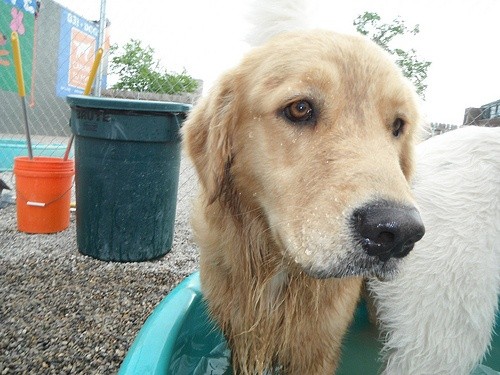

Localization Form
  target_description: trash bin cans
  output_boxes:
[63,95,192,264]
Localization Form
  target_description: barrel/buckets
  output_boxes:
[66,94,193,264]
[13,156,76,235]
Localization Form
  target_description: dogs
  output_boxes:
[180,28,427,375]
